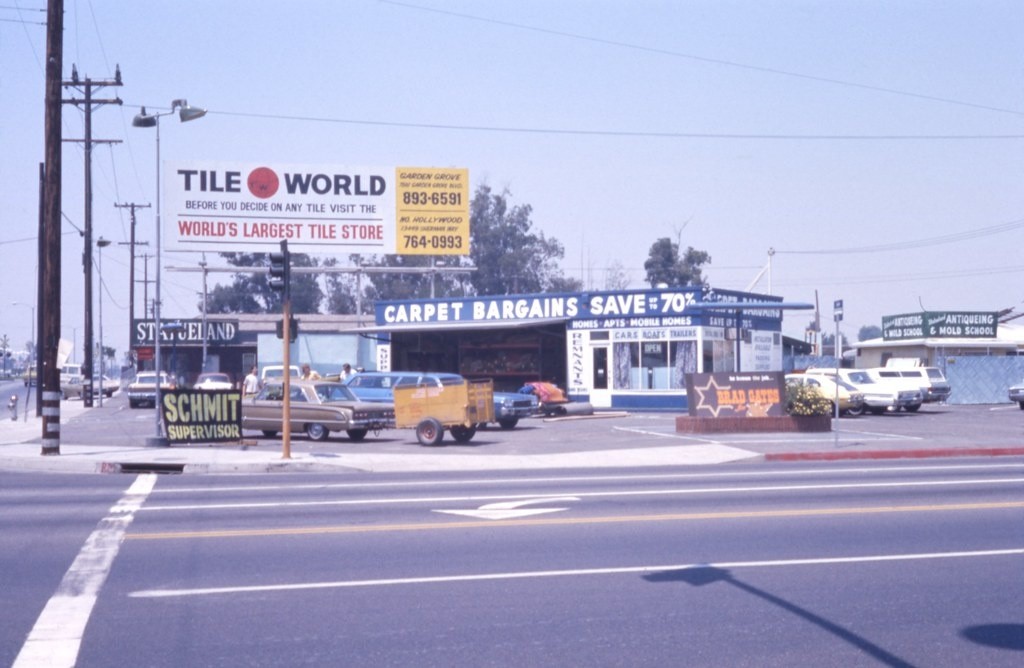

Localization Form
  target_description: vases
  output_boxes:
[787,413,836,432]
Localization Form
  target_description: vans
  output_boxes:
[60,363,89,384]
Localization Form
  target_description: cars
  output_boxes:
[59,375,118,400]
[191,371,235,395]
[126,370,174,407]
[22,364,37,388]
[1008,380,1024,411]
[241,380,393,440]
[784,373,864,416]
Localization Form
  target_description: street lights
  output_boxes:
[95,235,111,404]
[11,302,36,420]
[131,97,209,448]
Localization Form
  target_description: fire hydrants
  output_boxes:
[6,394,19,421]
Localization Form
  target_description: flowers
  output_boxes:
[784,376,834,417]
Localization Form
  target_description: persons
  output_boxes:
[300,363,321,382]
[339,363,361,387]
[519,384,536,394]
[357,368,368,385]
[242,365,260,396]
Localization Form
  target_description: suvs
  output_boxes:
[327,370,544,432]
[870,367,953,404]
[806,367,923,414]
